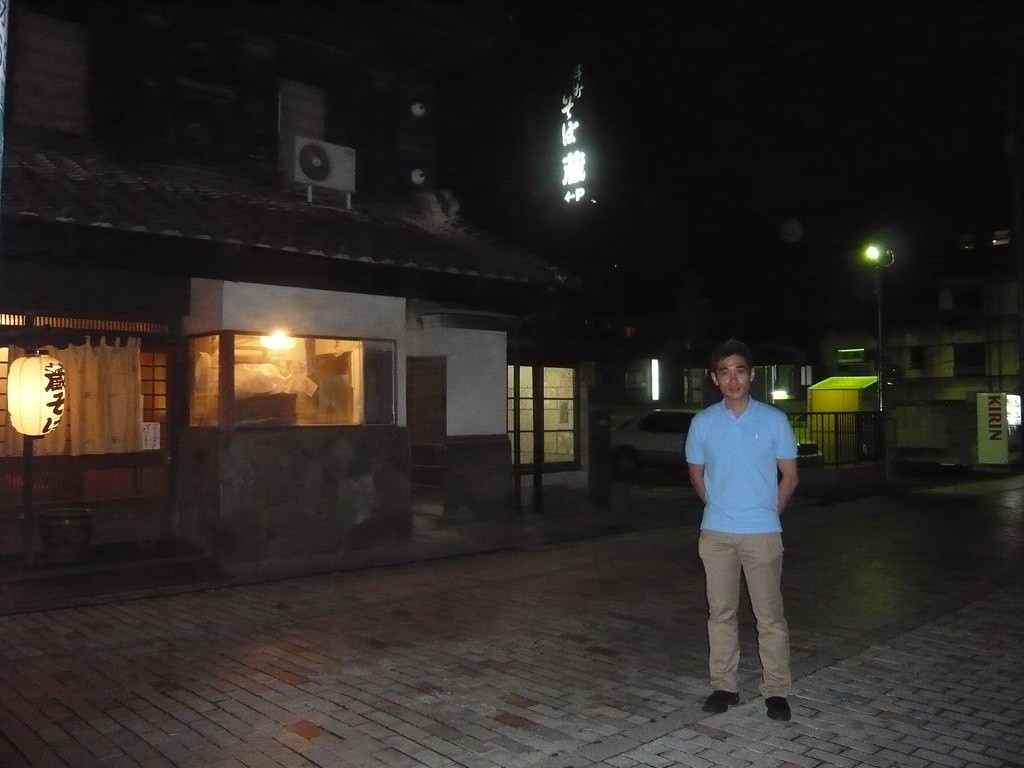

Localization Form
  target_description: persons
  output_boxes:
[685,339,799,720]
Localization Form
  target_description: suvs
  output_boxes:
[607,409,825,487]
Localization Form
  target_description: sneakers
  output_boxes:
[702,690,739,713]
[765,696,791,721]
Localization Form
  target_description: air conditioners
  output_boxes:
[289,134,355,195]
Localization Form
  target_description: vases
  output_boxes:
[39,505,93,561]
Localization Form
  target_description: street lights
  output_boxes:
[861,241,886,412]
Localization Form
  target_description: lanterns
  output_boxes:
[7,349,66,439]
